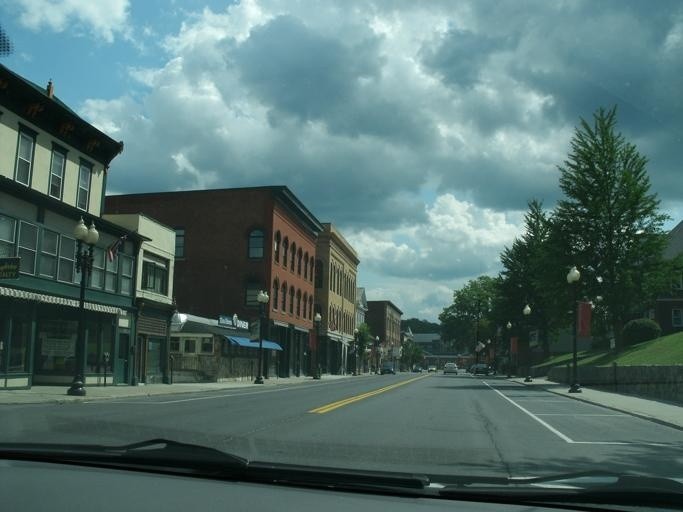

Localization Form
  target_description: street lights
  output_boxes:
[506,320,512,378]
[566,266,581,393]
[313,312,321,379]
[66,216,98,397]
[352,328,358,376]
[254,289,269,385]
[522,303,532,382]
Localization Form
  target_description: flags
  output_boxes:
[106,234,129,261]
[576,301,593,338]
[510,337,517,353]
[328,319,337,331]
[308,329,317,352]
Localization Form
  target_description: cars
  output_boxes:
[379,361,395,374]
[443,363,457,374]
[472,363,489,375]
[428,364,437,371]
[413,365,422,372]
